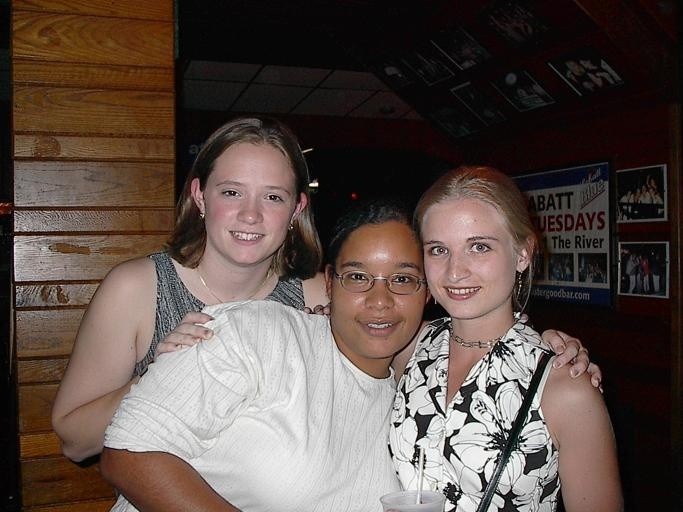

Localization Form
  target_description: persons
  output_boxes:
[104,200,609,512]
[49,115,340,502]
[299,164,630,512]
[617,174,666,297]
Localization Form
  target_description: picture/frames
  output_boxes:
[612,163,669,302]
[365,1,626,144]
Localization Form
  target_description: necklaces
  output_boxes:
[446,317,504,352]
[196,261,276,308]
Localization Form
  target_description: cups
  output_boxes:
[378,489,446,512]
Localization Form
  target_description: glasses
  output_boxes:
[329,266,427,295]
